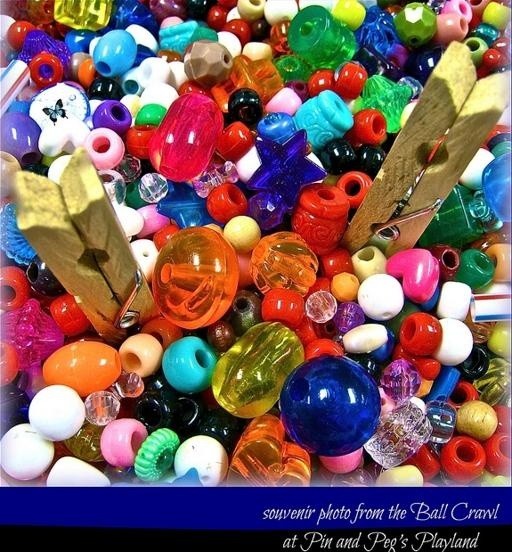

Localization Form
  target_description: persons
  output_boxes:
[0,1,512,485]
